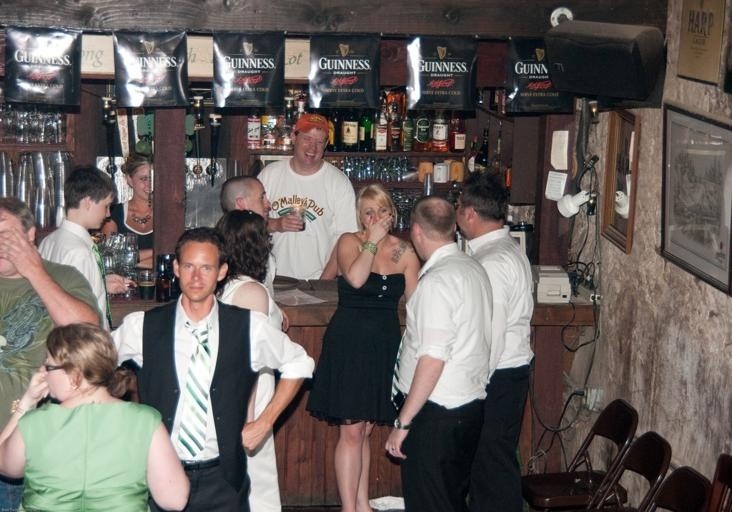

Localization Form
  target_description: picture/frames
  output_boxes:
[660,102,732,297]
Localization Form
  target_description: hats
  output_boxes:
[294,113,329,137]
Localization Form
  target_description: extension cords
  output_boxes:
[576,279,601,302]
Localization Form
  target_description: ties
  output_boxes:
[176,322,212,458]
[390,328,407,410]
[91,244,114,330]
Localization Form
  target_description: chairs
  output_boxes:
[519,397,638,512]
[647,466,711,512]
[590,431,672,512]
[703,455,732,512]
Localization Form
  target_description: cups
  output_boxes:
[98,233,140,299]
[138,270,157,300]
[339,156,462,230]
[2,107,67,231]
[157,252,177,301]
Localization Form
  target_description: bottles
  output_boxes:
[248,95,465,156]
[467,125,512,186]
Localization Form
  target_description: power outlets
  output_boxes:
[580,387,603,412]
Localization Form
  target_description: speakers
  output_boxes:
[545,20,664,101]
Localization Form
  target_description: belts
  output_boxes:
[496,365,530,374]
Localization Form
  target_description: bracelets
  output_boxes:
[364,241,378,254]
[13,407,28,416]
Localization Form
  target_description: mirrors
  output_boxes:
[600,109,642,257]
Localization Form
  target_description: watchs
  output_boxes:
[393,417,413,432]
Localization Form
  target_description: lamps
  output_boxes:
[556,154,598,218]
[614,190,631,220]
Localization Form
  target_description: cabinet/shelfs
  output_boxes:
[0,106,583,269]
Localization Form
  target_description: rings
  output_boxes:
[383,221,387,225]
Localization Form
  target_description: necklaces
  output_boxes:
[128,211,152,225]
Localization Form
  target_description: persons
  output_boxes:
[213,209,289,512]
[255,112,363,280]
[37,165,118,332]
[456,166,535,512]
[100,152,154,270]
[0,197,104,512]
[109,226,316,512]
[385,195,495,511]
[220,176,277,301]
[1,321,191,512]
[306,183,421,512]
